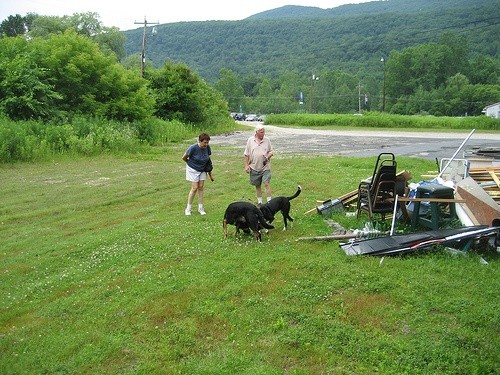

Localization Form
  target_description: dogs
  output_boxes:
[223,201,275,242]
[258,184,302,234]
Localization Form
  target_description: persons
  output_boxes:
[244,125,273,204]
[182,133,214,216]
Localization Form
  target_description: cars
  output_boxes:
[231,112,266,121]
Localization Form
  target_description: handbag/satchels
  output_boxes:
[198,159,213,172]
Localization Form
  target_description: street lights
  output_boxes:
[381,56,386,111]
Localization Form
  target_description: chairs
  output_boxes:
[357,153,397,223]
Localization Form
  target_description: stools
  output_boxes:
[411,183,456,232]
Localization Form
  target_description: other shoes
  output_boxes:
[198,208,206,215]
[257,203,263,208]
[185,209,191,215]
